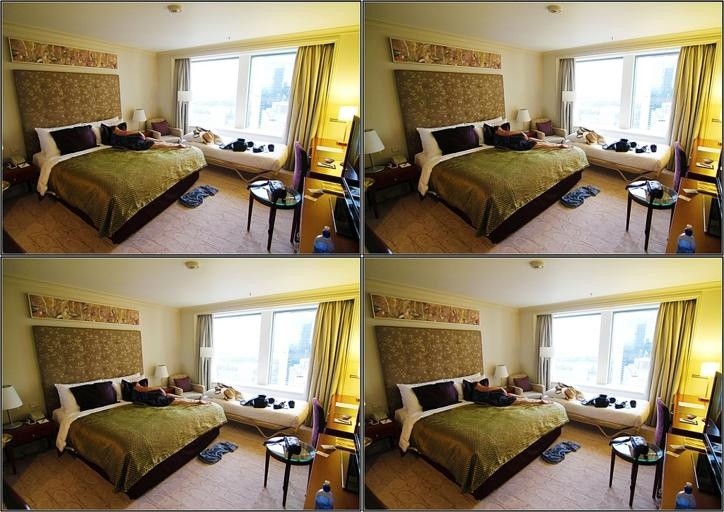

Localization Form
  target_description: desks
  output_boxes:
[665,138,721,254]
[661,394,721,510]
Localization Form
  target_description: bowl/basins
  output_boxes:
[323,158,334,164]
[340,414,351,421]
[703,158,713,164]
[685,414,697,420]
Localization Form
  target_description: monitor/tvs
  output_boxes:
[702,148,723,238]
[691,371,722,494]
[329,115,360,241]
[346,404,361,494]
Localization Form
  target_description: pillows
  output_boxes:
[183,130,206,143]
[152,120,171,136]
[37,114,128,161]
[415,116,511,161]
[568,131,589,144]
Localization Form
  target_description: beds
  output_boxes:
[626,180,678,251]
[32,141,590,511]
[562,129,672,183]
[543,389,651,440]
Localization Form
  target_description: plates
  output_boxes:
[334,417,353,426]
[317,161,336,170]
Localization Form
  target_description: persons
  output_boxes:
[108,124,188,152]
[491,123,569,152]
[469,380,552,407]
[128,380,209,407]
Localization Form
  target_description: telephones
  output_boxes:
[369,410,388,421]
[26,410,46,421]
[7,154,26,165]
[391,155,407,165]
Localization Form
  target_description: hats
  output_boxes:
[221,388,236,401]
[200,131,215,144]
[583,131,598,144]
[561,387,575,399]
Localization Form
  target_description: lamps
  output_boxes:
[698,361,720,402]
[516,109,531,132]
[364,129,386,175]
[131,109,147,133]
[336,104,356,146]
[3,384,24,430]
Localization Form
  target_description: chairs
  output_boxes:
[673,142,687,192]
[531,117,567,144]
[147,118,184,144]
[655,396,670,448]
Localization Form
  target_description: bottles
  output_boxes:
[314,480,334,509]
[675,481,698,509]
[313,226,335,253]
[676,225,697,254]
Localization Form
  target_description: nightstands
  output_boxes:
[3,157,36,201]
[3,415,55,474]
[524,129,537,138]
[143,131,153,137]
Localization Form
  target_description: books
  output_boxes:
[712,441,722,453]
[317,161,336,169]
[697,180,717,195]
[334,436,355,451]
[348,185,360,197]
[333,413,351,425]
[322,181,344,194]
[683,435,706,451]
